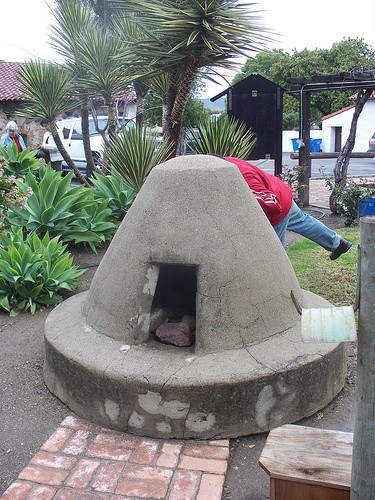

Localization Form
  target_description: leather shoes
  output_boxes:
[330,238,352,260]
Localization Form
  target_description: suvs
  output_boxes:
[43,116,141,180]
[182,127,207,155]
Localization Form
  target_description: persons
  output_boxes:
[0,121,27,153]
[209,154,352,260]
[38,118,65,172]
